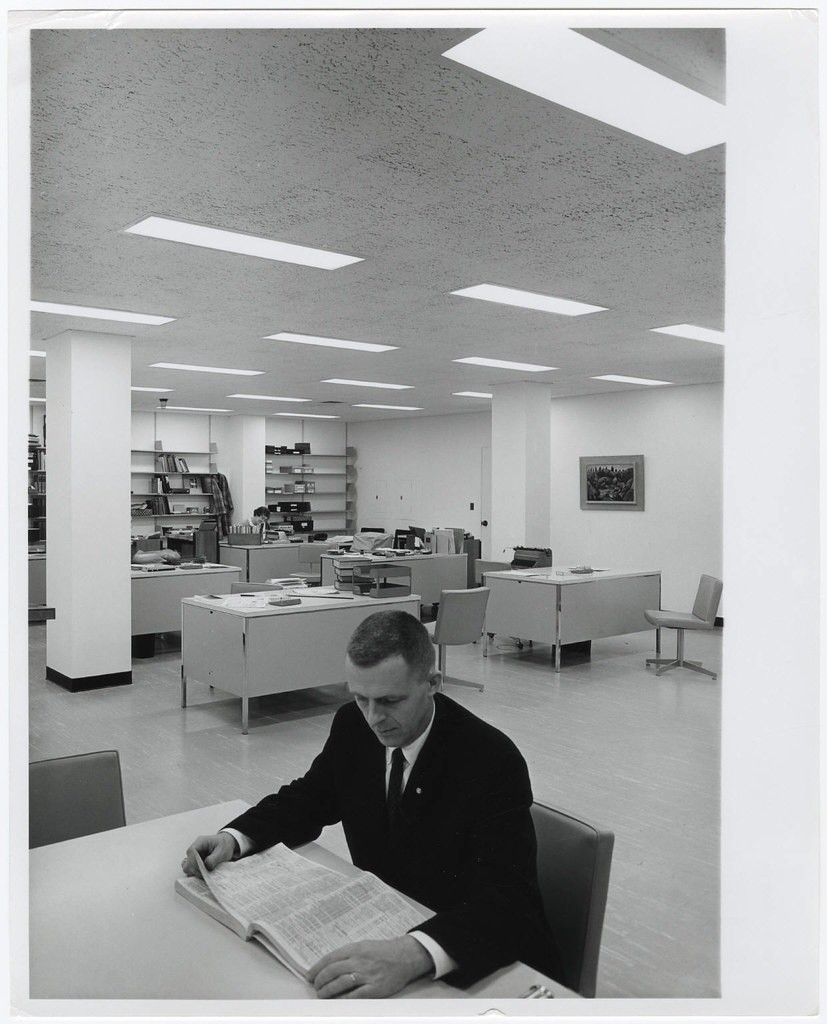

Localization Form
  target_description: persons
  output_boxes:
[180,610,552,999]
[131,516,180,563]
[240,506,271,540]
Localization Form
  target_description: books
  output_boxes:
[158,454,189,472]
[433,527,464,555]
[175,843,436,985]
[229,523,262,534]
[332,557,375,591]
[132,496,189,516]
[152,475,190,494]
[28,434,47,560]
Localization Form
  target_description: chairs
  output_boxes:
[424,587,490,692]
[30,750,125,848]
[474,558,524,649]
[291,543,332,587]
[643,574,723,680]
[230,583,283,596]
[349,532,393,551]
[531,799,615,999]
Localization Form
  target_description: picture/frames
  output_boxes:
[579,454,645,512]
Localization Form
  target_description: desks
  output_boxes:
[28,797,584,999]
[219,541,352,584]
[481,565,661,673]
[131,562,242,636]
[29,602,56,621]
[321,552,469,620]
[183,584,422,733]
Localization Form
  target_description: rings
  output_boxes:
[351,974,359,985]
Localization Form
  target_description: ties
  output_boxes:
[387,747,406,813]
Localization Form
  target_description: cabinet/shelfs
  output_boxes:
[129,440,218,519]
[28,447,46,606]
[268,452,356,533]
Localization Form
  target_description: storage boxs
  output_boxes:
[265,443,315,532]
[353,563,412,598]
